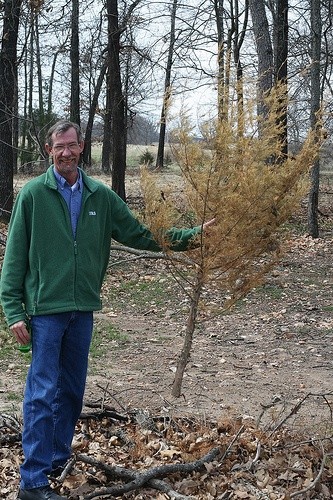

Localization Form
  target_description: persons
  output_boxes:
[0,119,216,500]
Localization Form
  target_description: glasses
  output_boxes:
[50,142,82,154]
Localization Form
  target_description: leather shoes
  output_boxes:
[19,485,66,500]
